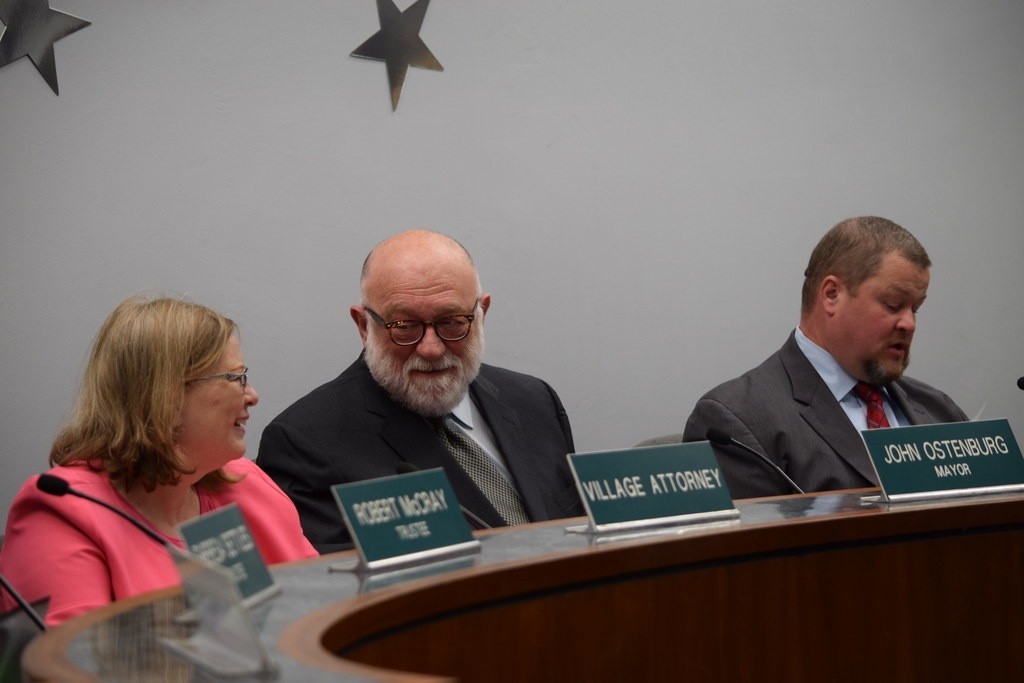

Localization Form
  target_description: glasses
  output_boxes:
[184,368,248,388]
[362,300,479,346]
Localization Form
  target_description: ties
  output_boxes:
[429,414,531,526]
[848,379,891,430]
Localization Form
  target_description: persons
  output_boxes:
[682,217,971,499]
[256,230,587,555]
[0,296,321,628]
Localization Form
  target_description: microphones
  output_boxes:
[397,463,493,531]
[1016,377,1024,391]
[706,427,805,496]
[36,474,169,547]
